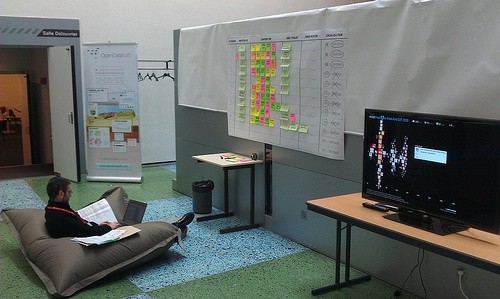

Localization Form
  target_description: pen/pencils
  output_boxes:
[220,153,251,164]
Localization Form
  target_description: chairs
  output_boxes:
[0,106,16,134]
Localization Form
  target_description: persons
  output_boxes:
[45,177,195,239]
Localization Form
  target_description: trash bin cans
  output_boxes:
[191,179,215,214]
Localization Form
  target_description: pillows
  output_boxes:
[0,185,185,299]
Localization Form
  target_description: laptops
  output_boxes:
[118,199,147,227]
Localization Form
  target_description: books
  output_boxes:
[76,198,117,225]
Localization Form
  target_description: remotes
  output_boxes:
[362,202,389,212]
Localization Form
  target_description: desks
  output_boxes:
[6,116,22,121]
[191,151,264,234]
[306,192,500,296]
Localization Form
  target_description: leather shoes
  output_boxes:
[172,212,195,229]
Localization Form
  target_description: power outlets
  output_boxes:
[454,260,467,281]
[300,208,308,223]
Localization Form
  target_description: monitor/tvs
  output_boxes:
[362,109,500,236]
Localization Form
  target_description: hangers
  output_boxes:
[138,70,174,82]
